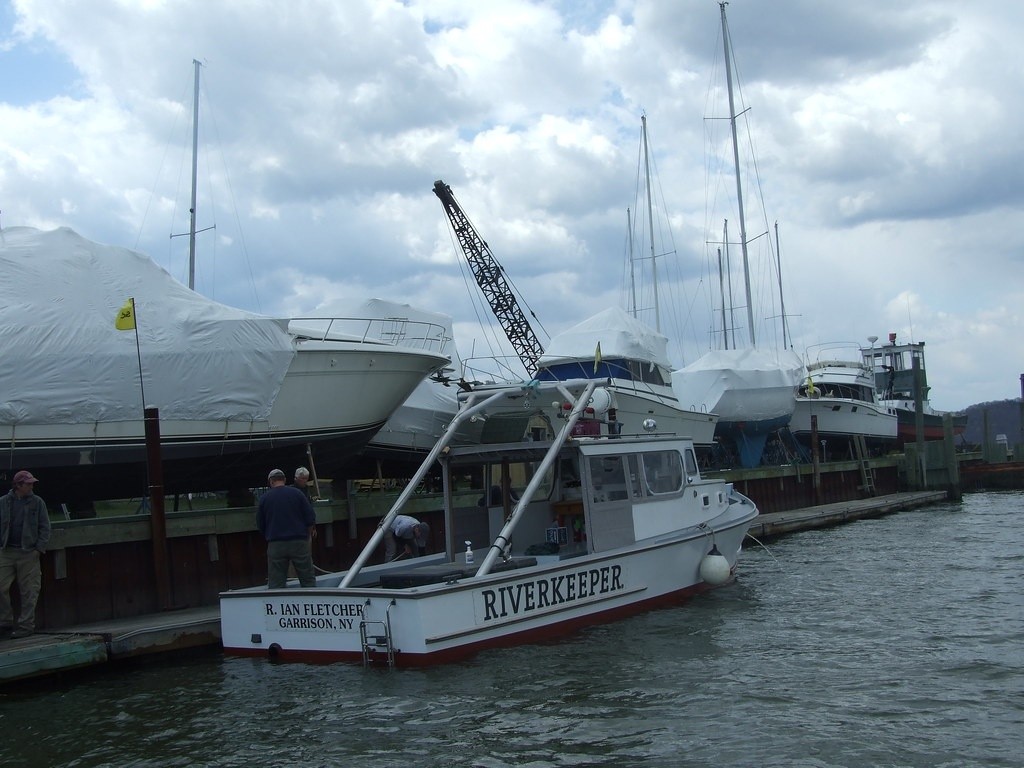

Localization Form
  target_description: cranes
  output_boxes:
[432,178,555,382]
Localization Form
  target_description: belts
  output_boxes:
[7,544,22,548]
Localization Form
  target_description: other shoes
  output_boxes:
[10,628,33,637]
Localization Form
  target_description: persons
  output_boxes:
[287,465,312,579]
[383,516,430,563]
[256,469,317,590]
[476,476,520,506]
[0,471,50,638]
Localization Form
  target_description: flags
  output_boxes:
[594,343,602,374]
[808,377,815,394]
[116,299,136,332]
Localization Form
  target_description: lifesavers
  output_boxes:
[807,386,821,399]
[556,514,586,541]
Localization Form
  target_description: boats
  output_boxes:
[0,292,971,522]
[217,351,761,675]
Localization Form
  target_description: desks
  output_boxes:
[554,501,584,527]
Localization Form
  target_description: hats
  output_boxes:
[419,522,430,541]
[14,471,40,483]
[268,469,284,480]
